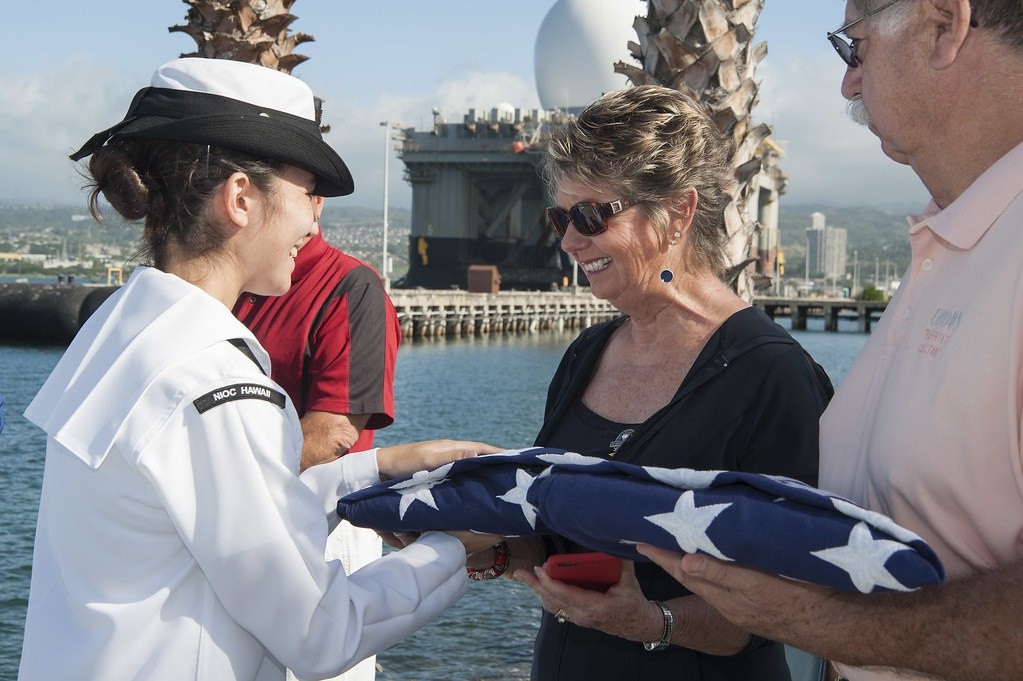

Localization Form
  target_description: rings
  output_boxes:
[554,608,568,624]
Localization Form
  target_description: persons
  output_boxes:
[377,85,834,681]
[637,1,1023,681]
[15,56,506,681]
[232,194,402,681]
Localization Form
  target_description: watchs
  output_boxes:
[640,600,674,652]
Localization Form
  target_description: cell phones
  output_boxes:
[545,552,623,594]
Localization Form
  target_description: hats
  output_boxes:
[68,57,355,197]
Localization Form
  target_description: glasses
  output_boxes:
[828,0,979,68]
[545,195,643,238]
